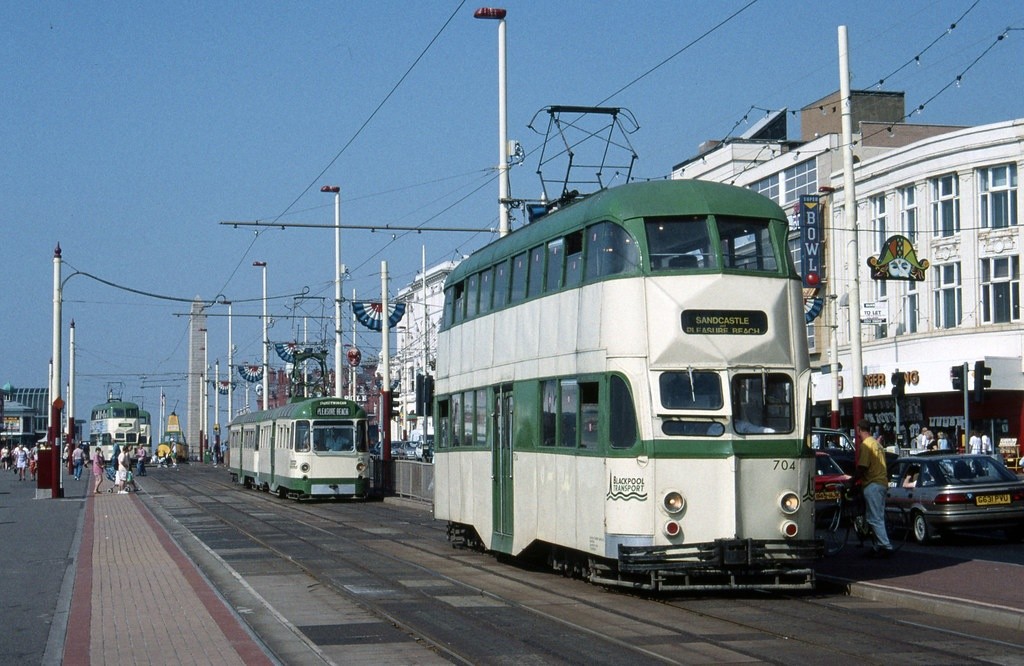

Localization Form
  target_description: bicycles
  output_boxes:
[816,483,916,559]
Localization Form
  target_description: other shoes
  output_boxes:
[75,476,77,480]
[874,549,894,560]
[94,491,100,494]
[134,488,141,491]
[19,479,22,481]
[863,551,876,559]
[117,489,129,494]
[77,478,79,480]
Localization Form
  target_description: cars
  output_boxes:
[882,453,1024,545]
[366,426,434,462]
[812,446,852,517]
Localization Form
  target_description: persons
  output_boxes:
[325,430,352,450]
[134,444,147,476]
[213,441,220,465]
[0,443,51,480]
[92,439,142,494]
[968,430,991,454]
[154,438,177,467]
[849,421,893,550]
[706,419,775,435]
[903,464,937,488]
[911,428,954,450]
[64,441,89,480]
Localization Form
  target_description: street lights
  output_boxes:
[218,300,234,423]
[320,184,343,398]
[198,328,209,452]
[252,261,269,411]
[472,7,512,238]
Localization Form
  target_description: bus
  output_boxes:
[434,103,826,597]
[131,395,153,464]
[226,347,373,500]
[87,380,141,466]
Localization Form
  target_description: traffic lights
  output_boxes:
[891,371,907,397]
[950,363,965,390]
[390,389,402,417]
[974,360,992,403]
